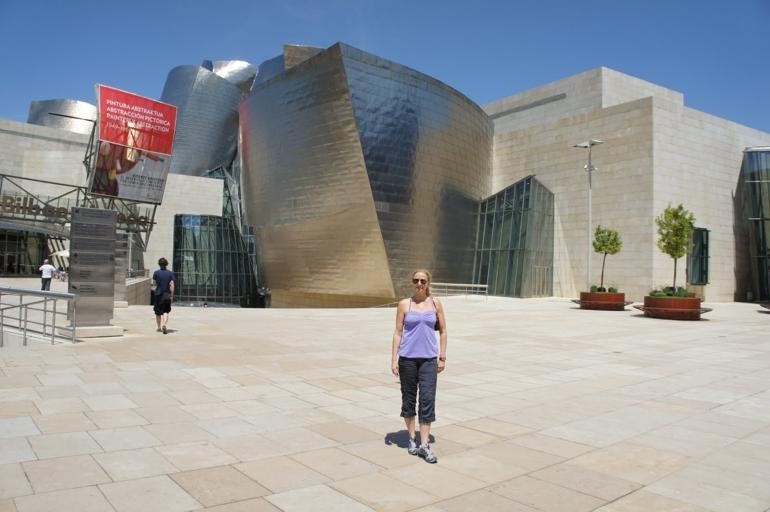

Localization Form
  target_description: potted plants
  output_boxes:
[643,201,701,320]
[581,223,624,311]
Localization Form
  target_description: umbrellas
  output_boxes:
[48,249,70,257]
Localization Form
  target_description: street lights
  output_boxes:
[572,138,606,293]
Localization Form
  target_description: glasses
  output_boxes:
[413,279,426,284]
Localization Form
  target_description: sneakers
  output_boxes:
[157,326,167,334]
[408,438,437,463]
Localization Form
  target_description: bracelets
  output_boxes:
[170,296,174,297]
[439,357,446,361]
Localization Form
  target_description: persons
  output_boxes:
[151,258,175,334]
[391,269,447,463]
[56,267,69,281]
[39,259,56,291]
